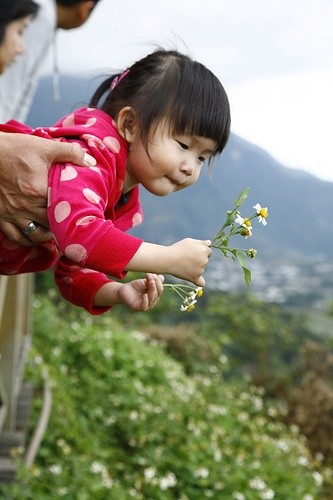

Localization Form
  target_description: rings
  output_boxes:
[22,221,40,237]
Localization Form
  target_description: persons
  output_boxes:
[0,51,232,316]
[0,0,102,247]
